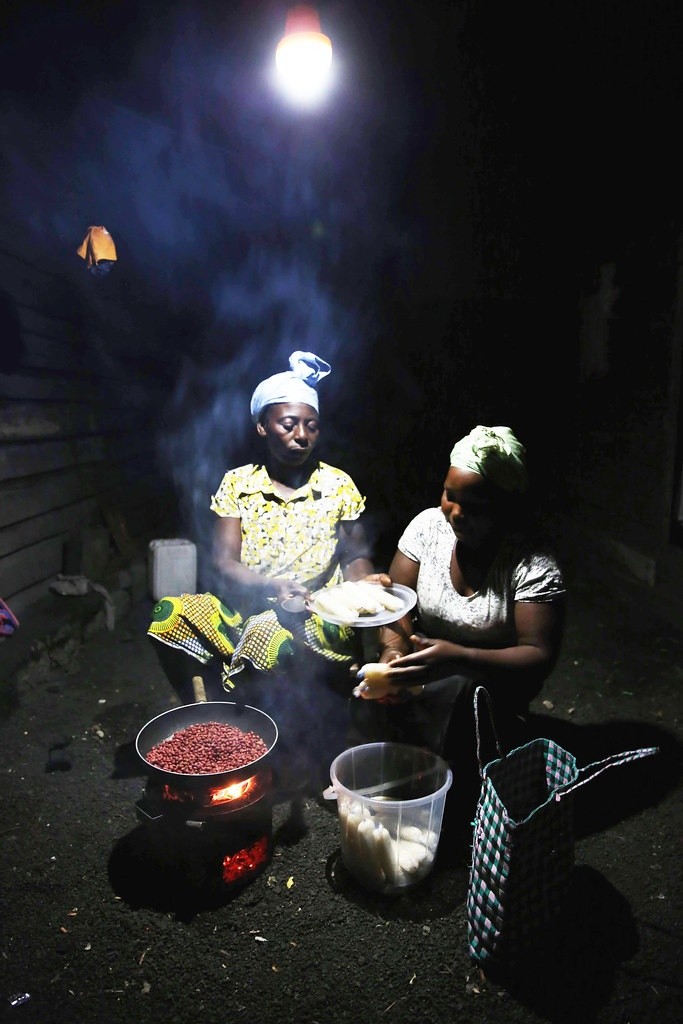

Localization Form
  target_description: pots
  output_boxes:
[133,674,279,800]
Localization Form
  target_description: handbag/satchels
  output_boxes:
[467,684,662,966]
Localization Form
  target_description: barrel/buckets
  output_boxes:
[322,740,454,893]
[147,536,198,602]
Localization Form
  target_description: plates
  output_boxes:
[305,579,418,629]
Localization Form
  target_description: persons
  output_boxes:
[323,424,567,801]
[147,350,392,794]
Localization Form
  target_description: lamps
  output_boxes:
[273,0,335,107]
